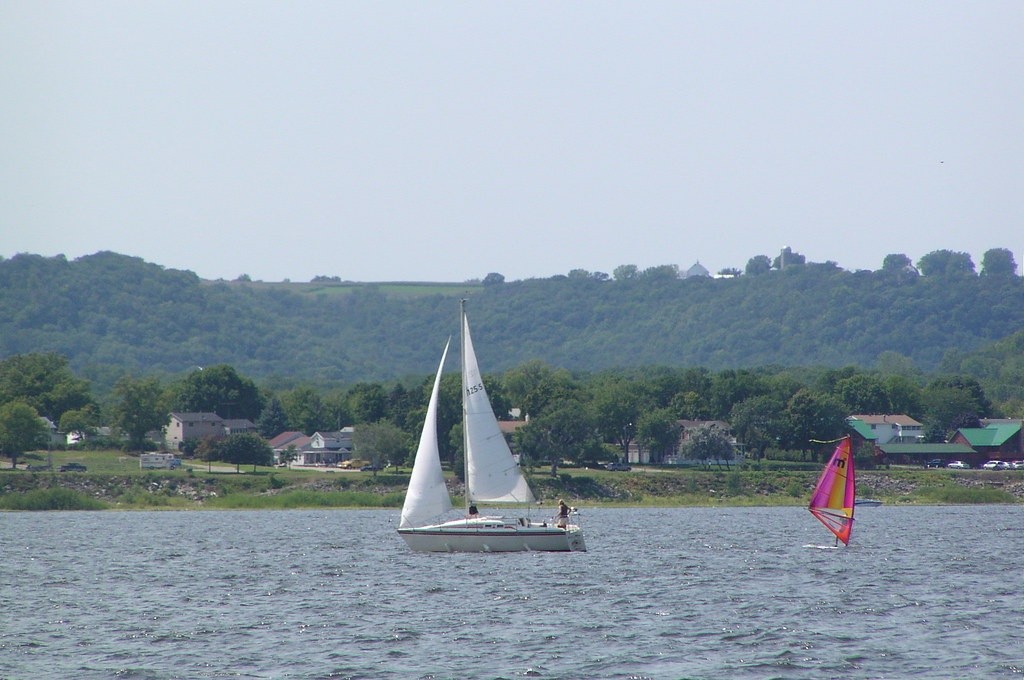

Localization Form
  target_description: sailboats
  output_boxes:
[801,434,856,549]
[396,295,589,553]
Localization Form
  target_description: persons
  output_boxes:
[469,501,478,519]
[552,500,571,530]
[835,513,847,547]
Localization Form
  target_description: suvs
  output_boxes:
[607,462,632,472]
[927,458,946,468]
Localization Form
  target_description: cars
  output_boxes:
[947,461,970,469]
[983,460,1024,471]
[360,462,383,471]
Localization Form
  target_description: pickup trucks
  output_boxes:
[341,457,369,470]
[57,462,87,472]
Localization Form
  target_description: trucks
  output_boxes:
[139,454,175,469]
